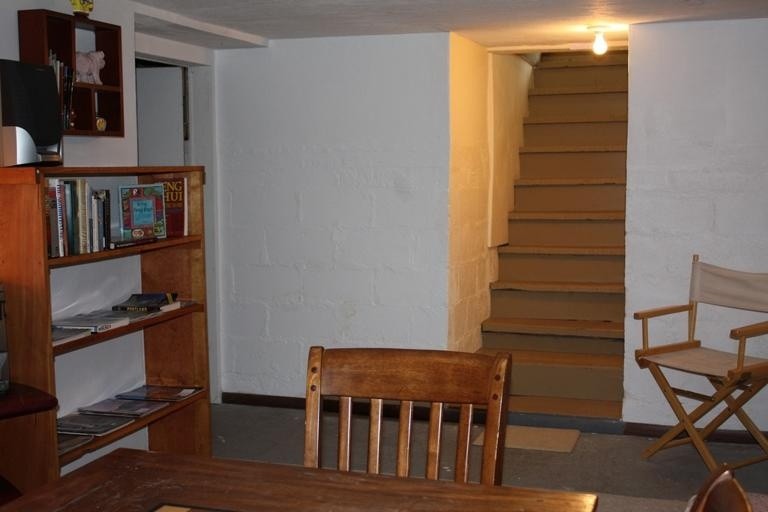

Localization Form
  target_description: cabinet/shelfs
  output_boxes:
[15,10,126,139]
[1,163,212,500]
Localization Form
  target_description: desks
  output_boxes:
[0,446,599,512]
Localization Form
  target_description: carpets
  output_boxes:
[472,417,581,455]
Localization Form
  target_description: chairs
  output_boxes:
[632,253,768,477]
[298,342,510,489]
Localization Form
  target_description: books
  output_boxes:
[57,385,204,457]
[52,293,180,347]
[48,49,76,129]
[44,177,190,258]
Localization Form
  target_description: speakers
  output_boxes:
[0,60,63,168]
